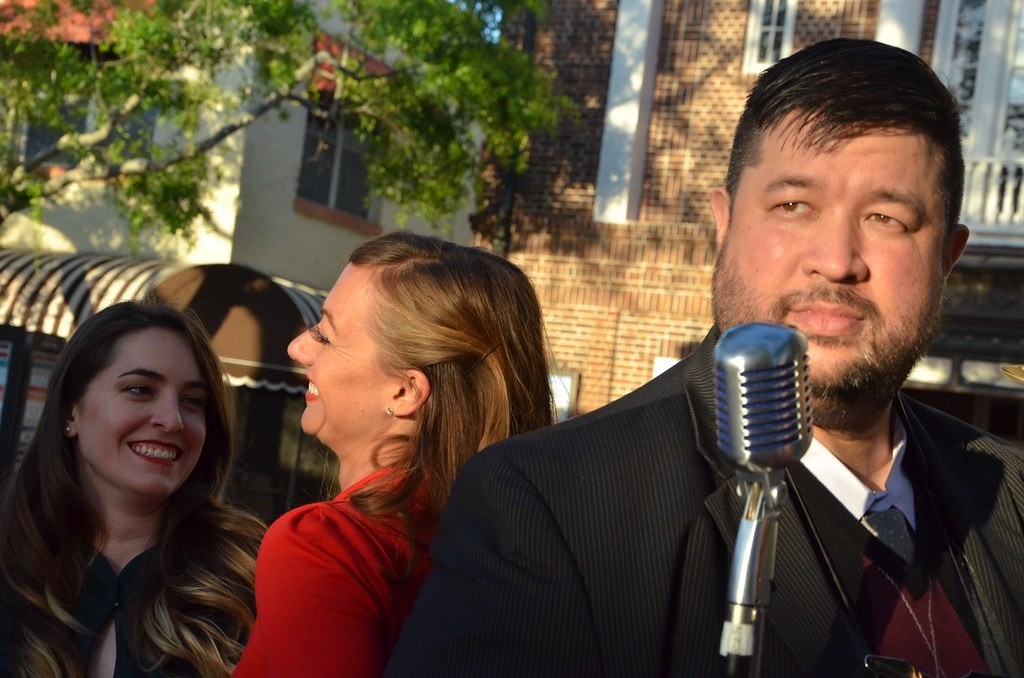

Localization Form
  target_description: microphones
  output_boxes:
[711,320,813,678]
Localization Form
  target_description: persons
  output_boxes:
[391,36,1024,678]
[0,298,267,678]
[231,228,558,678]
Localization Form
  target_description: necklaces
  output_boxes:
[876,566,949,678]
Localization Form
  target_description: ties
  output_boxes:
[860,503,912,566]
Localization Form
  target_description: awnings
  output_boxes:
[0,245,326,395]
